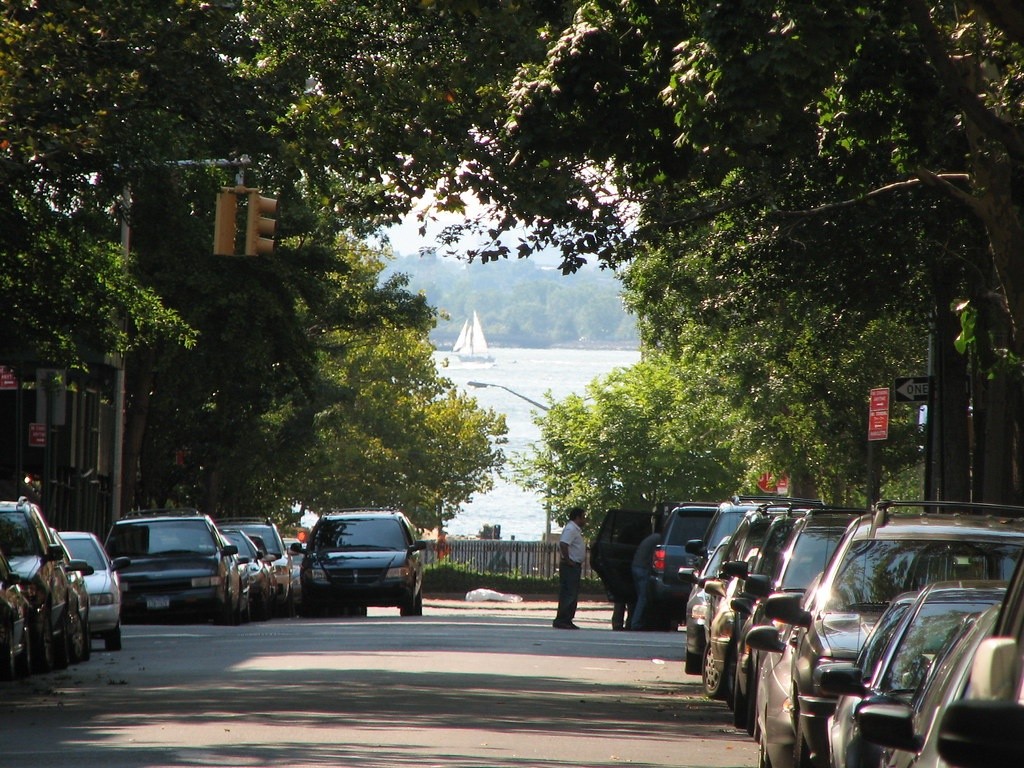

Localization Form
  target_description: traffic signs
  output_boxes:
[893,375,927,402]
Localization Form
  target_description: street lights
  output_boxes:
[468,380,554,543]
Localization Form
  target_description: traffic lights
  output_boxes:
[244,192,278,257]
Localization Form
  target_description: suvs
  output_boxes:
[0,497,92,679]
[290,506,429,617]
[587,498,720,621]
[679,495,1023,768]
[101,508,245,627]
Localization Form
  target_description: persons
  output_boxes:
[553,507,586,629]
[612,532,662,631]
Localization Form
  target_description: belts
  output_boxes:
[561,558,581,564]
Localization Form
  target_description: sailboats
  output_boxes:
[451,312,496,365]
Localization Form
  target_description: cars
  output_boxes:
[209,515,310,619]
[57,529,131,652]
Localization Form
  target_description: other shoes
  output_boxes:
[626,625,631,630]
[569,621,580,629]
[553,619,573,629]
[613,626,625,630]
